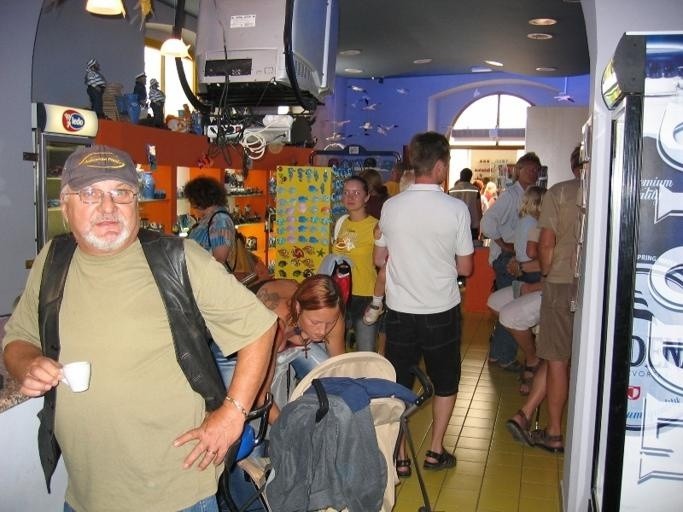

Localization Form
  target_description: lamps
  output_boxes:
[86,0,125,17]
[159,0,192,59]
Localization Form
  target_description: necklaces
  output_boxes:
[292,309,313,360]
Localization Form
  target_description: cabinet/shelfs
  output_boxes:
[221,168,268,267]
[175,163,224,234]
[136,164,174,231]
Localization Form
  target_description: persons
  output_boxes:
[0,143,282,512]
[84,57,105,118]
[332,132,584,478]
[148,79,166,128]
[179,180,245,275]
[210,278,347,439]
[132,73,148,122]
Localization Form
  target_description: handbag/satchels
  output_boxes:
[207,210,257,286]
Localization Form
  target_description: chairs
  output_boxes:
[220,392,276,511]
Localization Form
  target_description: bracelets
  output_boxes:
[224,395,249,425]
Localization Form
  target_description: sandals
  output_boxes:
[396,458,411,476]
[531,429,564,452]
[506,409,536,448]
[424,447,456,468]
[520,357,544,396]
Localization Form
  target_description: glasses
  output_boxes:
[341,190,360,198]
[64,189,140,204]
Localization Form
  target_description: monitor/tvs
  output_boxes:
[194,0,340,105]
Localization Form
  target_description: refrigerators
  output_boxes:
[27,99,99,256]
[590,29,683,511]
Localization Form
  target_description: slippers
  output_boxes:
[498,360,523,372]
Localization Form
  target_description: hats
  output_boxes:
[60,144,140,192]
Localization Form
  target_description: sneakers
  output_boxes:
[362,301,385,326]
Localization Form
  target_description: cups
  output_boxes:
[60,361,90,394]
